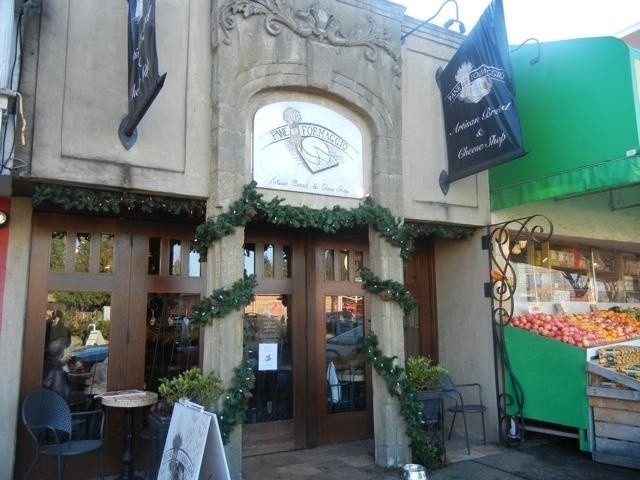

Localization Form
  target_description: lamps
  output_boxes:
[400,0,466,46]
[511,38,541,65]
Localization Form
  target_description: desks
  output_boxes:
[101,389,158,479]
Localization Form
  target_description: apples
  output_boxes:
[590,313,638,339]
[508,312,606,347]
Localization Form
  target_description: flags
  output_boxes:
[124,0,162,135]
[439,0,525,185]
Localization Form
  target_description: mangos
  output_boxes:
[605,324,625,342]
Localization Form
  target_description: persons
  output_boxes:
[45,310,72,372]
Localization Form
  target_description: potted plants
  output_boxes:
[150,365,225,480]
[405,354,448,425]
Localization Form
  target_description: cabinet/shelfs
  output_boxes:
[508,232,640,303]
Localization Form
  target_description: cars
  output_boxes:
[325,324,364,370]
[68,344,110,374]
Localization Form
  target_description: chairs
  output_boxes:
[21,387,107,480]
[429,371,486,455]
